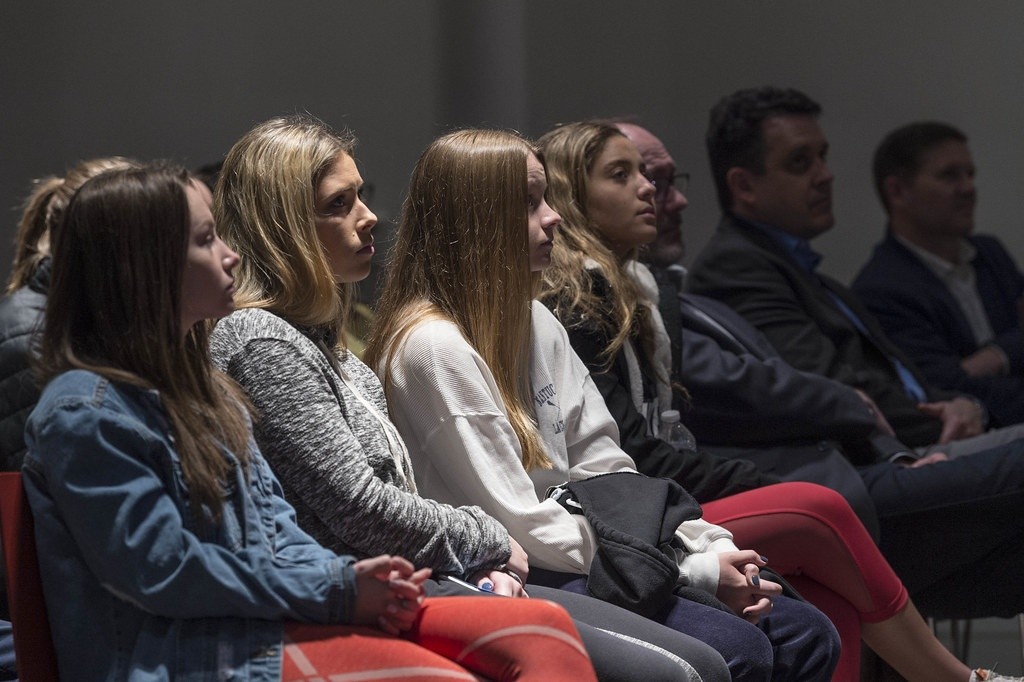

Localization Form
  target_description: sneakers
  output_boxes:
[968,665,1022,682]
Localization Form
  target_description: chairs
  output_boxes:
[0,472,58,682]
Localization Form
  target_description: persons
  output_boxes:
[358,125,843,682]
[200,114,735,682]
[20,161,602,681]
[528,120,1024,682]
[844,121,1024,431]
[1,157,234,482]
[605,124,1024,620]
[689,83,1024,449]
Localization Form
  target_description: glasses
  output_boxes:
[652,167,692,201]
